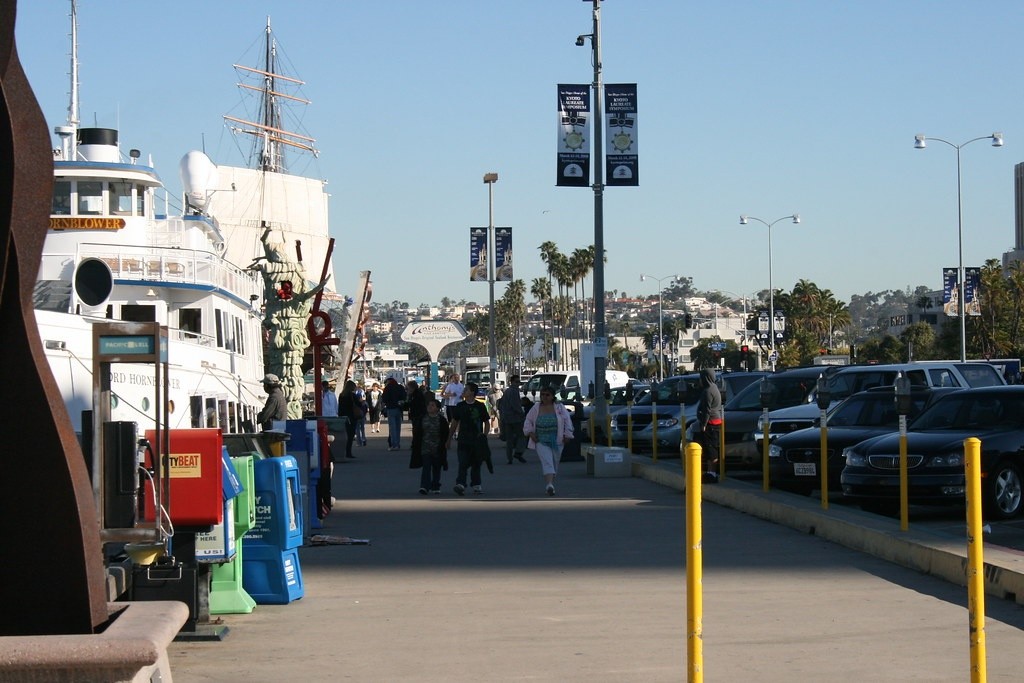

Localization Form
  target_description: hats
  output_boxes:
[259,373,281,385]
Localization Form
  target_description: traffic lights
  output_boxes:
[741,345,750,361]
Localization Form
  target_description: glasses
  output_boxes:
[463,387,471,392]
[540,392,552,397]
[514,380,520,384]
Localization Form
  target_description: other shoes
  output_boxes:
[544,483,557,497]
[418,487,429,496]
[377,428,381,433]
[355,443,361,447]
[346,453,355,457]
[362,441,367,446]
[507,459,513,464]
[513,452,527,463]
[370,428,376,434]
[702,472,719,484]
[431,490,441,495]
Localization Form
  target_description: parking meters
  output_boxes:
[893,369,912,531]
[815,370,831,510]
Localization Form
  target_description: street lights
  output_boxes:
[707,288,783,346]
[914,132,1004,363]
[738,213,801,372]
[640,273,681,382]
[480,172,502,391]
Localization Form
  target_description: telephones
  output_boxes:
[101,420,158,493]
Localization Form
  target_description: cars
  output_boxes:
[840,385,1024,521]
[601,357,1022,471]
[358,378,381,387]
[769,385,1003,496]
[438,369,534,397]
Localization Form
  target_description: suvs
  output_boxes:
[753,362,1008,461]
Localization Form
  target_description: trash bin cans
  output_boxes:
[142,427,220,622]
[194,444,244,622]
[243,453,305,604]
[209,455,257,614]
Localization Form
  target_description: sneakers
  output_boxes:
[387,446,401,452]
[472,484,483,492]
[453,484,466,496]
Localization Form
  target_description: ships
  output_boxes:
[24,0,347,488]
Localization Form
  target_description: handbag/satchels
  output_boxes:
[708,417,723,427]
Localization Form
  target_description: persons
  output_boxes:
[256,374,288,457]
[320,373,573,496]
[696,369,723,484]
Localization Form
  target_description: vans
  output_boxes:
[517,370,630,406]
